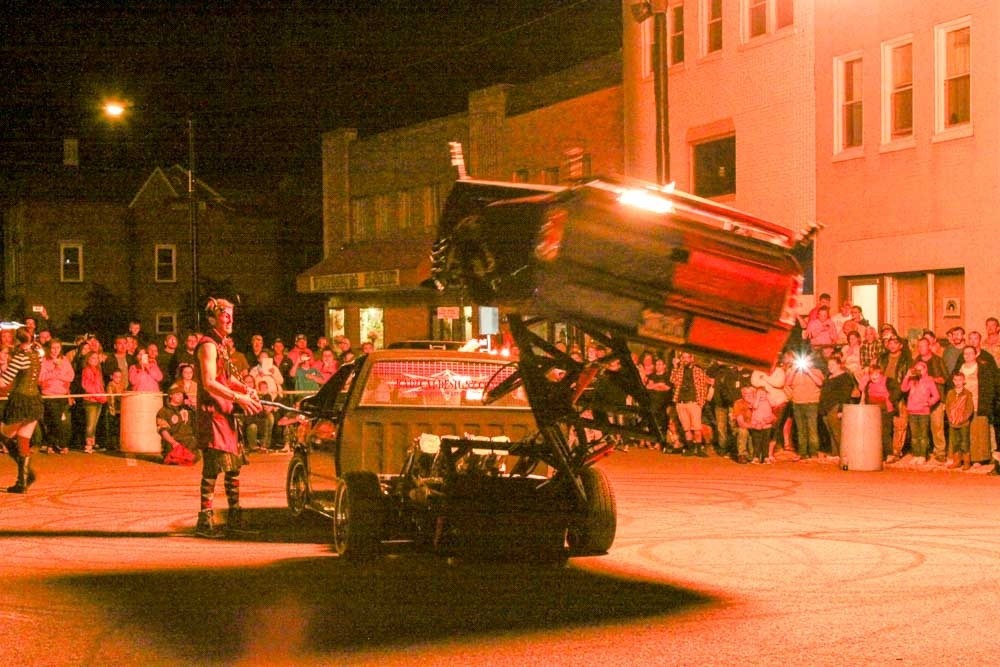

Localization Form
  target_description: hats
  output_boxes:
[169,388,189,400]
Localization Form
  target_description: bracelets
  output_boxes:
[232,393,239,402]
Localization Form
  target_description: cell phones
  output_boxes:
[32,306,43,312]
[911,370,921,374]
[141,354,148,364]
[301,356,309,360]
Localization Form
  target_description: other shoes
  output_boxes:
[84,444,92,454]
[917,456,927,465]
[60,447,69,455]
[886,455,896,463]
[718,447,728,456]
[935,455,946,464]
[973,461,982,468]
[750,457,760,464]
[909,455,919,464]
[47,447,55,454]
[763,457,771,465]
[738,455,747,464]
[988,462,1000,475]
[948,463,962,469]
[963,463,971,470]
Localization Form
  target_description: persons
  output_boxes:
[191,301,263,537]
[157,332,374,464]
[0,317,163,454]
[0,327,42,492]
[553,332,825,463]
[792,292,1000,475]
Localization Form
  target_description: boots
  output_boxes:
[196,510,225,537]
[8,440,35,486]
[223,508,263,534]
[7,456,30,493]
[684,440,695,456]
[696,443,709,457]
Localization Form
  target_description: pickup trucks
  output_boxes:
[279,140,830,557]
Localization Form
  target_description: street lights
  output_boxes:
[632,0,671,185]
[97,94,201,340]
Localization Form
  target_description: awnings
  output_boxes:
[295,240,436,292]
